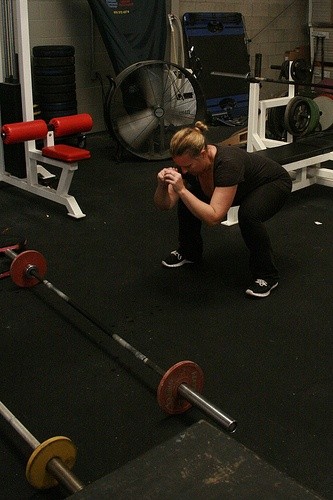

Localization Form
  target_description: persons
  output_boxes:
[151,120,293,298]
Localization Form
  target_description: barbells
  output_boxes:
[4,246,240,433]
[1,400,84,493]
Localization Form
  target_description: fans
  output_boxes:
[104,59,207,160]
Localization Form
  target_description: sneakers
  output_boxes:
[246,273,279,298]
[162,250,196,267]
